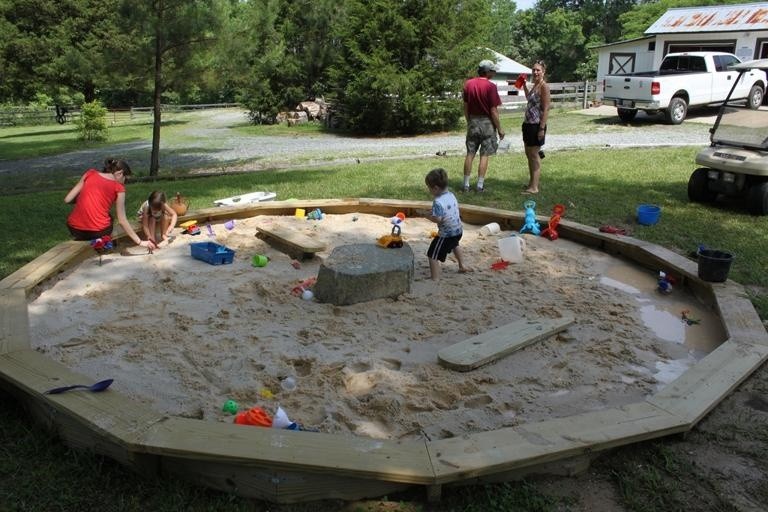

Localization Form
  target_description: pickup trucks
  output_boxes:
[600,50,767,125]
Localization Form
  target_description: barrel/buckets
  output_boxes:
[696,246,737,283]
[636,204,662,225]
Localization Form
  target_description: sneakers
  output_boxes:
[463,185,488,193]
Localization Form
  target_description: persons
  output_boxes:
[63,157,156,249]
[459,60,505,193]
[516,60,551,195]
[422,167,474,282]
[137,190,178,247]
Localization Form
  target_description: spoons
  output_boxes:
[43,378,114,399]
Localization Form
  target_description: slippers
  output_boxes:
[521,190,534,194]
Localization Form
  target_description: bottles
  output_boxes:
[697,239,705,255]
[392,217,403,225]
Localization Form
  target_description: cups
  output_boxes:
[515,71,525,90]
[252,254,268,267]
[295,208,306,218]
[225,220,235,230]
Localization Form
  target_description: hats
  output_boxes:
[478,60,500,70]
